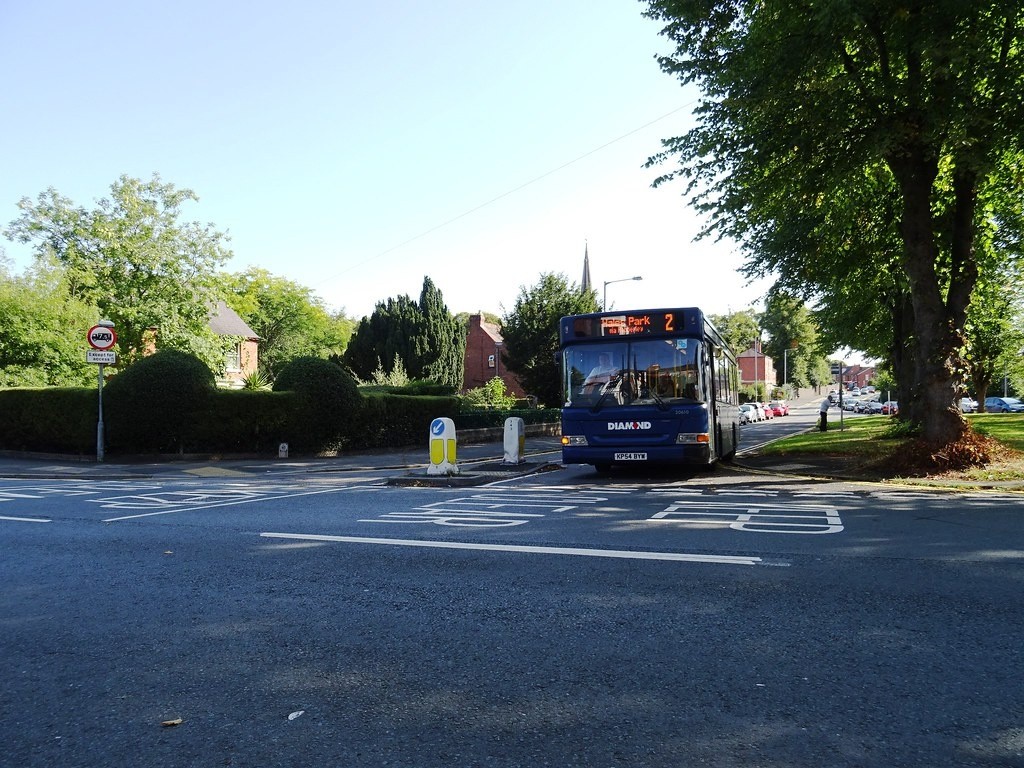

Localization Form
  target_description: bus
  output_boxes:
[555,306,740,477]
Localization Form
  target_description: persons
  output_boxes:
[615,371,641,398]
[653,375,675,397]
[820,396,839,432]
[585,354,618,383]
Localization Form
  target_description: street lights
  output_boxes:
[782,346,798,385]
[602,275,644,311]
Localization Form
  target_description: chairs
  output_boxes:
[652,379,676,398]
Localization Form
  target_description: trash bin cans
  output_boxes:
[499,417,527,464]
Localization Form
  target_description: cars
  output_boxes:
[961,398,980,413]
[829,384,898,415]
[738,406,748,426]
[740,405,758,423]
[779,402,789,416]
[770,403,784,417]
[984,396,1024,413]
[762,403,773,420]
[743,402,766,420]
